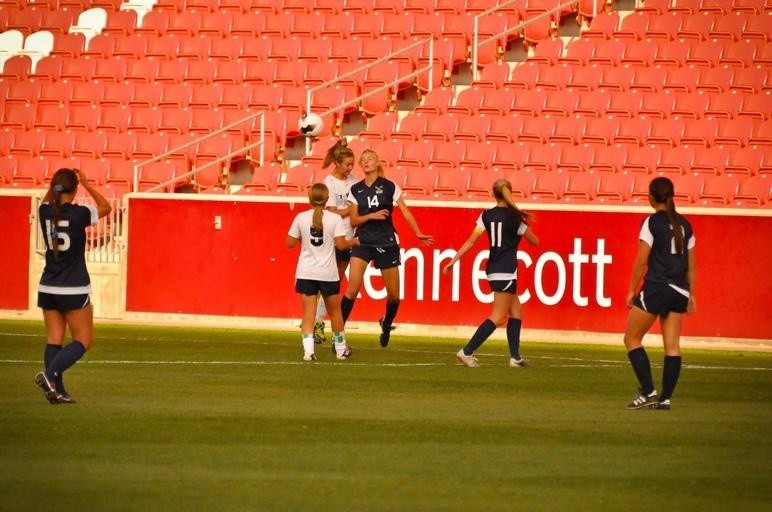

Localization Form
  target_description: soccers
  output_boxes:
[298,114,324,136]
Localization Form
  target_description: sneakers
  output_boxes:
[299,322,326,361]
[379,317,391,346]
[332,338,352,360]
[510,356,529,368]
[626,390,658,410]
[650,398,670,410]
[457,349,480,368]
[34,372,74,403]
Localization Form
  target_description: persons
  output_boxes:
[34,168,112,405]
[443,179,540,367]
[332,149,437,353]
[298,138,358,345]
[623,177,697,410]
[286,183,362,361]
